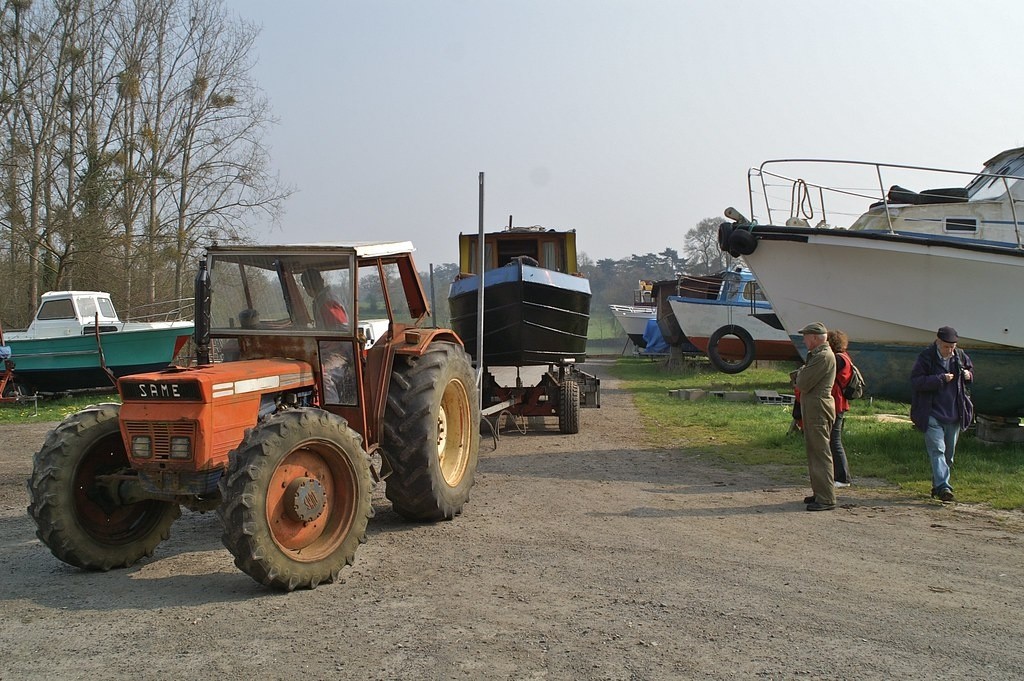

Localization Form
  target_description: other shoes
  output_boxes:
[804,496,835,511]
[931,488,953,500]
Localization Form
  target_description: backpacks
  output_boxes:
[834,352,866,400]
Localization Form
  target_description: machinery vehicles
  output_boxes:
[26,242,482,593]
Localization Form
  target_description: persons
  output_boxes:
[792,330,852,488]
[301,268,354,404]
[911,326,973,500]
[795,322,836,511]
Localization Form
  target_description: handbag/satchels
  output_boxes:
[957,347,976,426]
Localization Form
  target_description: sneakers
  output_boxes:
[834,482,850,487]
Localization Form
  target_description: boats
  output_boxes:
[0,290,195,399]
[446,215,591,367]
[666,264,803,362]
[717,147,1024,417]
[609,290,701,353]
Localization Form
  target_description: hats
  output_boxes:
[797,322,827,334]
[937,326,958,343]
